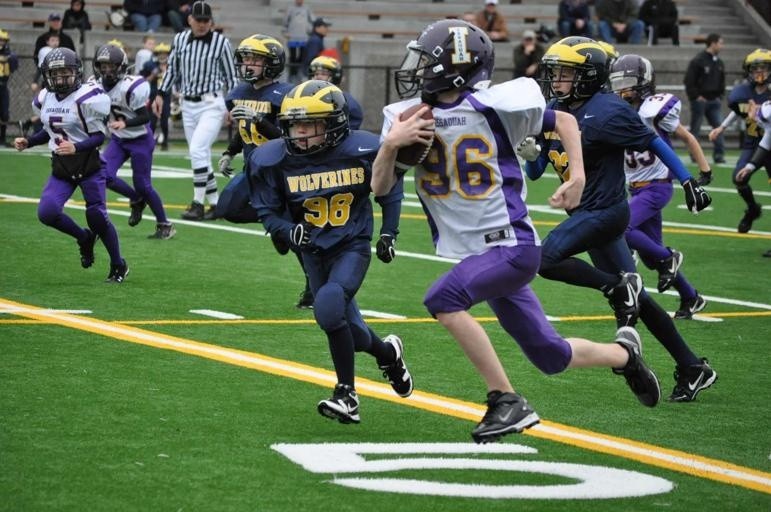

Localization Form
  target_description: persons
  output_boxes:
[282,1,332,82]
[218,18,718,441]
[473,0,680,46]
[684,34,771,257]
[1,0,240,282]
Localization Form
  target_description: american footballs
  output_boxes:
[394,104,433,177]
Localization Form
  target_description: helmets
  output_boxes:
[536,36,655,103]
[233,33,349,157]
[41,44,128,93]
[742,48,771,86]
[395,19,495,99]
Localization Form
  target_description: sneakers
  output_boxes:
[376,334,414,398]
[182,201,223,220]
[147,223,177,240]
[77,228,99,268]
[104,258,129,284]
[471,389,540,444]
[738,204,762,234]
[317,384,360,424]
[604,247,718,407]
[128,195,146,226]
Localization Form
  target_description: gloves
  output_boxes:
[288,222,320,254]
[683,170,714,216]
[376,233,396,263]
[229,105,262,124]
[218,154,235,178]
[516,137,541,162]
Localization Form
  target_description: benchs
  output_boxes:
[1,0,710,44]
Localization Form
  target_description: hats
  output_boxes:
[192,1,212,20]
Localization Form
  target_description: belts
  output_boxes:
[184,93,217,101]
